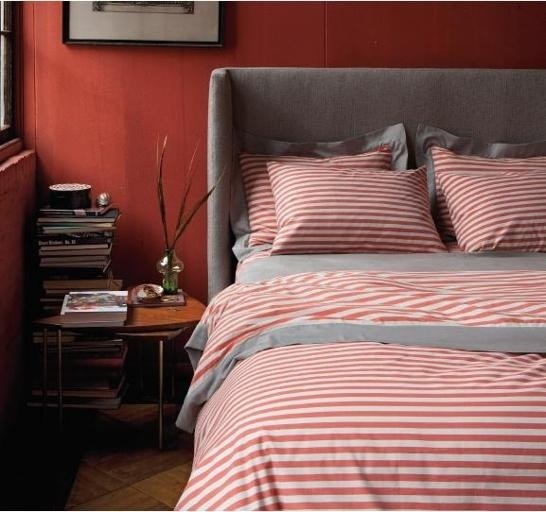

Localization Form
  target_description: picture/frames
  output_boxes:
[60,2,227,49]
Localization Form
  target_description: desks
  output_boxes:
[33,281,208,453]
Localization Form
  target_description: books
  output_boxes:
[26,204,128,409]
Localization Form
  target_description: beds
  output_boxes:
[199,66,546,510]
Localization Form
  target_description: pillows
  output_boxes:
[228,122,449,264]
[414,123,546,262]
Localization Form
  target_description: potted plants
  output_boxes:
[153,134,228,295]
